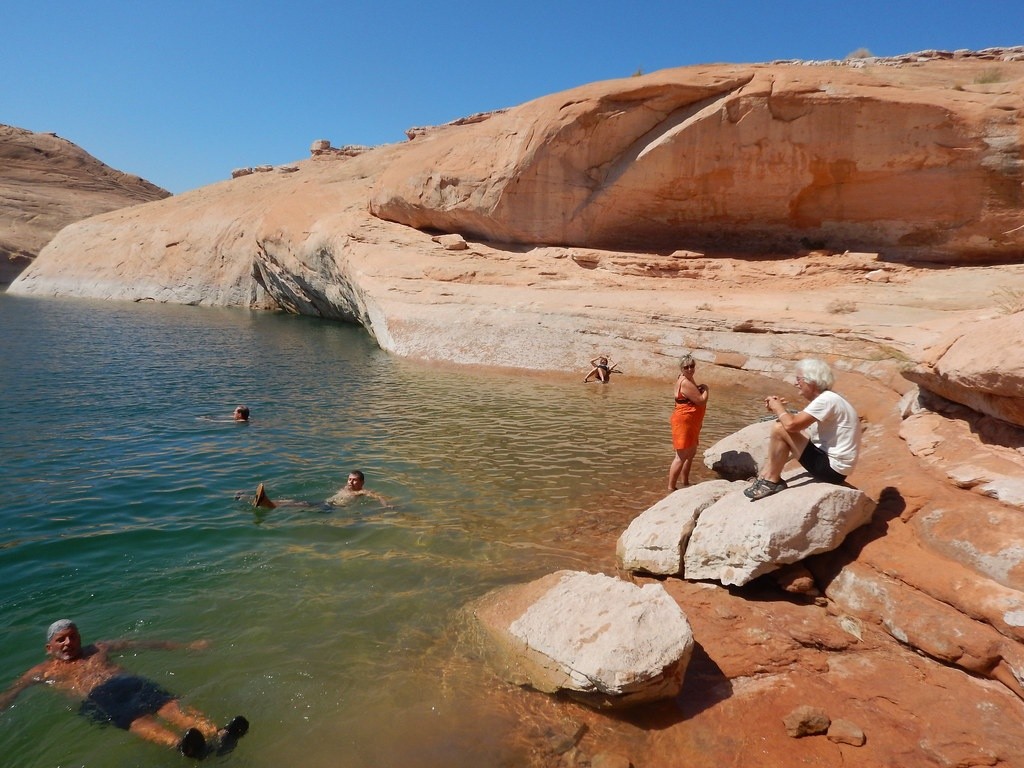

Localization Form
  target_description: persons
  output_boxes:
[744,358,859,499]
[195,406,249,422]
[0,619,249,759]
[583,355,617,384]
[252,471,400,511]
[667,355,708,491]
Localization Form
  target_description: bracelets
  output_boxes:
[778,412,788,417]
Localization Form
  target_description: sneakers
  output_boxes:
[744,478,787,498]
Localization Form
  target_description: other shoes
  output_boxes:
[214,715,249,758]
[251,483,266,509]
[581,378,587,384]
[601,380,605,384]
[177,728,214,763]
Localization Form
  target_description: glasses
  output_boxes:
[682,364,695,370]
